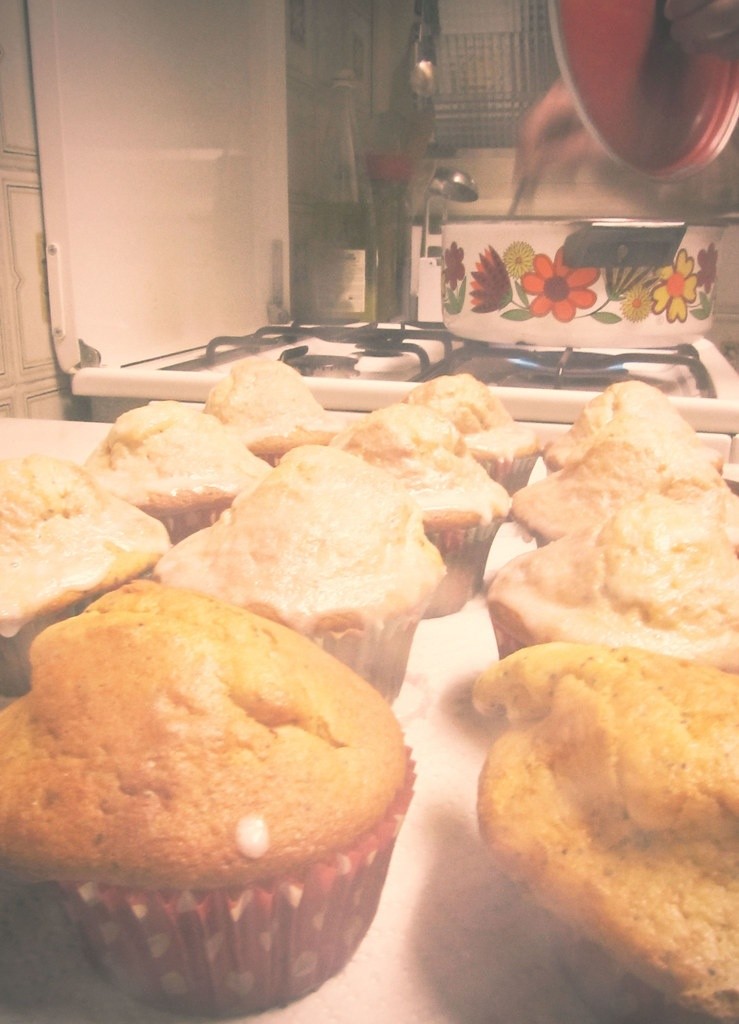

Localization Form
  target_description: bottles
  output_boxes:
[417,245,442,323]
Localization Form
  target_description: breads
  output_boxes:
[0,360,739,1024]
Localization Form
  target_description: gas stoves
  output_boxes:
[158,323,738,435]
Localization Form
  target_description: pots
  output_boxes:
[440,219,729,348]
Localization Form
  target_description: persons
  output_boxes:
[511,0,739,185]
